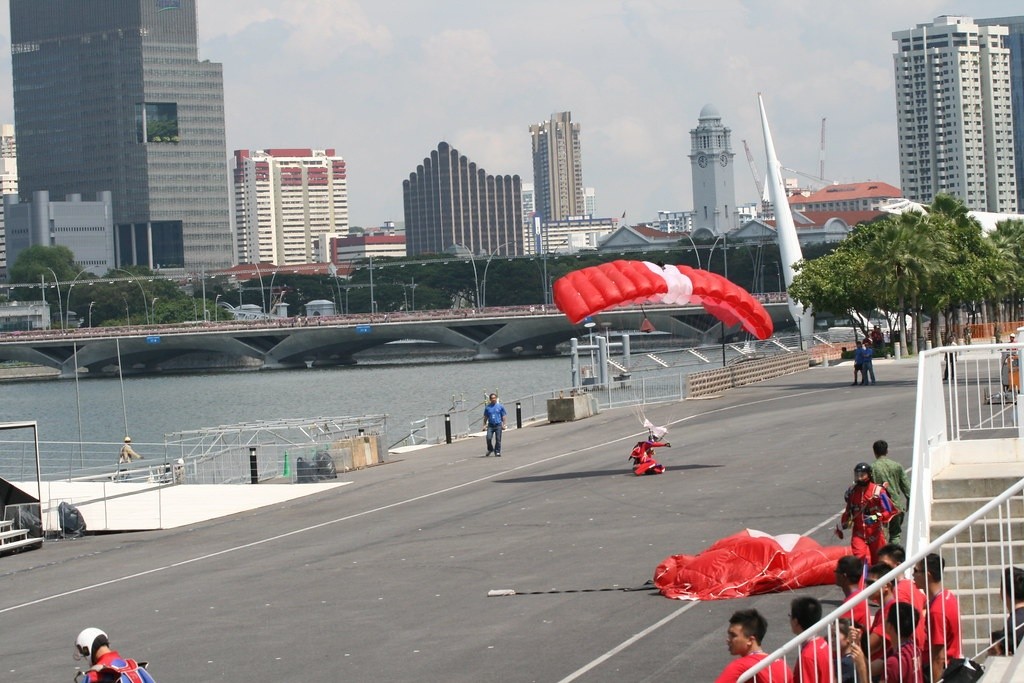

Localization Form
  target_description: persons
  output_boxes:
[175,458,186,481]
[628,434,672,476]
[963,322,974,345]
[530,304,546,315]
[278,317,322,327]
[715,543,1024,683]
[993,319,1003,343]
[1008,333,1016,343]
[483,393,508,457]
[849,325,884,386]
[841,440,911,569]
[943,335,958,380]
[111,436,143,480]
[370,313,387,323]
[464,309,476,319]
[73,628,154,683]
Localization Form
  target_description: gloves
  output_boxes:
[864,514,878,525]
[666,443,672,448]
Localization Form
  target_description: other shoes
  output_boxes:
[495,452,501,456]
[486,449,493,457]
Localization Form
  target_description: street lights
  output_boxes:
[548,241,566,305]
[114,269,149,325]
[346,254,360,314]
[89,301,94,330]
[483,240,517,305]
[460,243,480,313]
[29,305,32,331]
[519,247,545,304]
[42,267,63,328]
[680,233,700,267]
[707,233,723,273]
[152,297,158,326]
[67,266,98,329]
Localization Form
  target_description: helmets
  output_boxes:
[76,628,108,666]
[854,463,872,486]
[1010,333,1015,337]
[125,437,131,442]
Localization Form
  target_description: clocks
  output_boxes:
[720,155,727,167]
[698,156,707,168]
